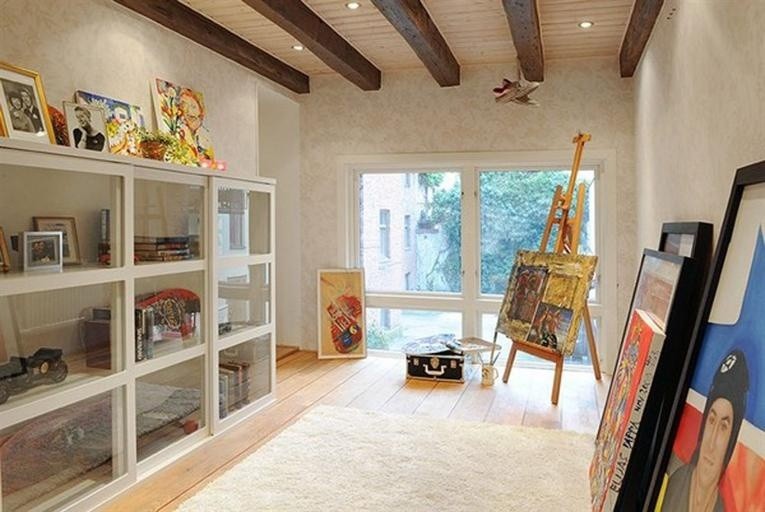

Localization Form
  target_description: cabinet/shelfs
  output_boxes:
[0,139,277,512]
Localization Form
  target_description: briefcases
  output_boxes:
[405,336,502,384]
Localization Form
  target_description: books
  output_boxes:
[100,208,112,264]
[84,307,154,370]
[218,360,251,419]
[134,234,194,262]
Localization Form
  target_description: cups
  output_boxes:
[481,364,499,386]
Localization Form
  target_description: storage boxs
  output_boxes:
[406,350,466,383]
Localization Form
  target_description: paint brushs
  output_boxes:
[477,351,501,364]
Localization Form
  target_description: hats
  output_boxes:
[697,350,747,468]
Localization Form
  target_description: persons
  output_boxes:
[72,106,105,152]
[31,241,52,262]
[8,86,44,134]
[660,349,749,511]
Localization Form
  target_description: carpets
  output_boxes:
[181,404,597,511]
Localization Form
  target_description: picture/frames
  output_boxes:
[650,159,765,511]
[317,268,365,359]
[0,59,57,143]
[62,101,111,152]
[596,220,714,510]
